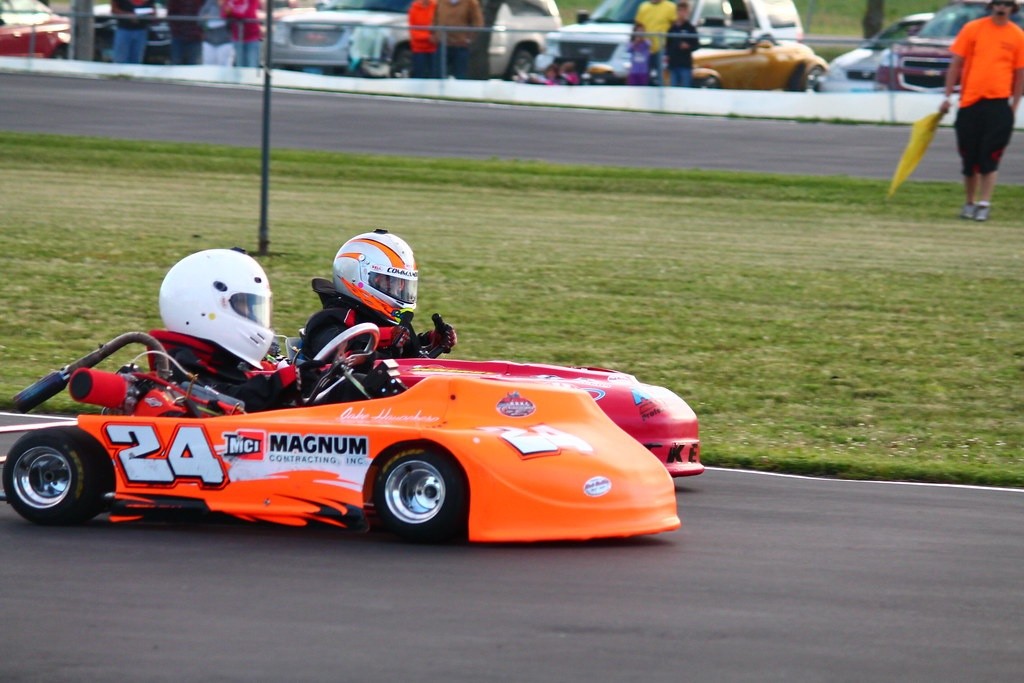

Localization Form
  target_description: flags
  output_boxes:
[886,113,939,198]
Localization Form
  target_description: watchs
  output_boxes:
[941,96,949,101]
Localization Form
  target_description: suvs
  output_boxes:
[873,0,1024,94]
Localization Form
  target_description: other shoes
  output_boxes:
[975,202,990,221]
[961,203,974,218]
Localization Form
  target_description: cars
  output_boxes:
[1,0,270,65]
[263,0,561,83]
[806,13,956,93]
[534,0,803,81]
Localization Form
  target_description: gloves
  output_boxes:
[428,314,457,353]
[296,359,326,395]
[391,325,411,348]
[351,349,376,374]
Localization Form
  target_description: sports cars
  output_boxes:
[587,24,831,88]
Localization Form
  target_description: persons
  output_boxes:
[111,0,262,68]
[624,0,696,88]
[407,0,483,79]
[303,230,457,402]
[158,248,375,418]
[938,0,1024,221]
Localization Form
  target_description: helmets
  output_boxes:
[160,250,275,370]
[333,232,418,320]
